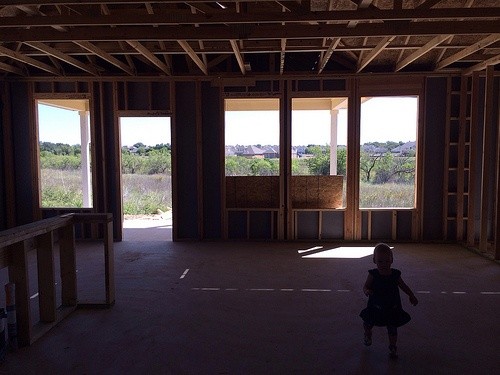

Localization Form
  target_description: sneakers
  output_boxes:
[388,345,399,358]
[363,332,372,346]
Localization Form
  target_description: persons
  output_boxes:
[362,242,419,356]
